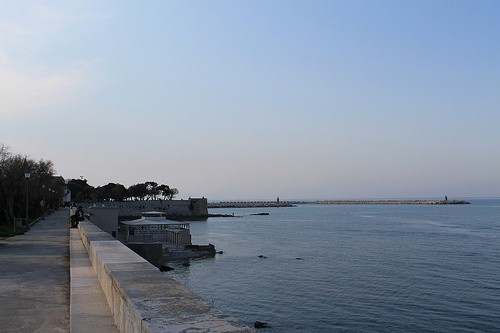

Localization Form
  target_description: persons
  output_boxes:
[64,197,205,228]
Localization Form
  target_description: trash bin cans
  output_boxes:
[16,218,22,228]
[25,219,30,225]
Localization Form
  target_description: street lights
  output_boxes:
[24,169,57,227]
